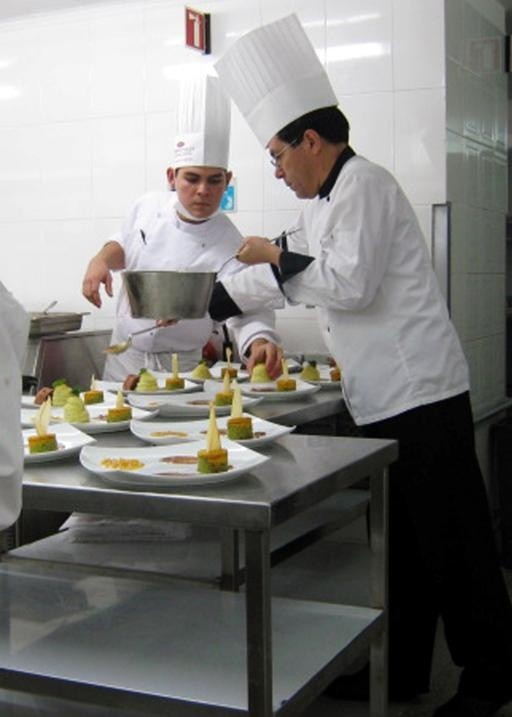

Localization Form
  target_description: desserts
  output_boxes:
[26,344,342,475]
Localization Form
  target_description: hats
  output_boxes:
[214,13,339,149]
[175,75,230,171]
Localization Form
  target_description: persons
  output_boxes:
[212,15,512,717]
[82,76,283,383]
[0,273,32,532]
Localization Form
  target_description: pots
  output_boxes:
[120,229,301,320]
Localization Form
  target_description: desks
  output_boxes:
[6,365,397,592]
[0,424,399,717]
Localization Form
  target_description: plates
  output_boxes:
[78,436,268,485]
[123,388,264,417]
[22,359,340,407]
[22,401,158,434]
[129,413,297,449]
[22,423,96,465]
